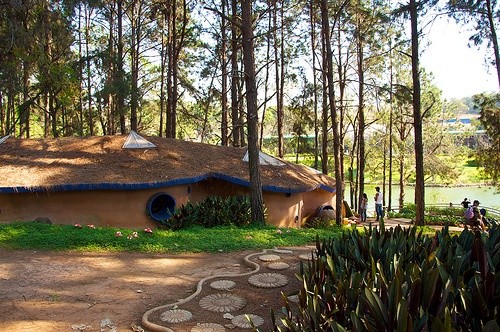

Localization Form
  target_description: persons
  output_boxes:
[480,209,492,231]
[461,197,471,209]
[359,194,369,222]
[466,200,489,232]
[374,187,384,222]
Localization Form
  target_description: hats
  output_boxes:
[474,200,481,204]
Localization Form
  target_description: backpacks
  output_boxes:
[463,206,476,219]
